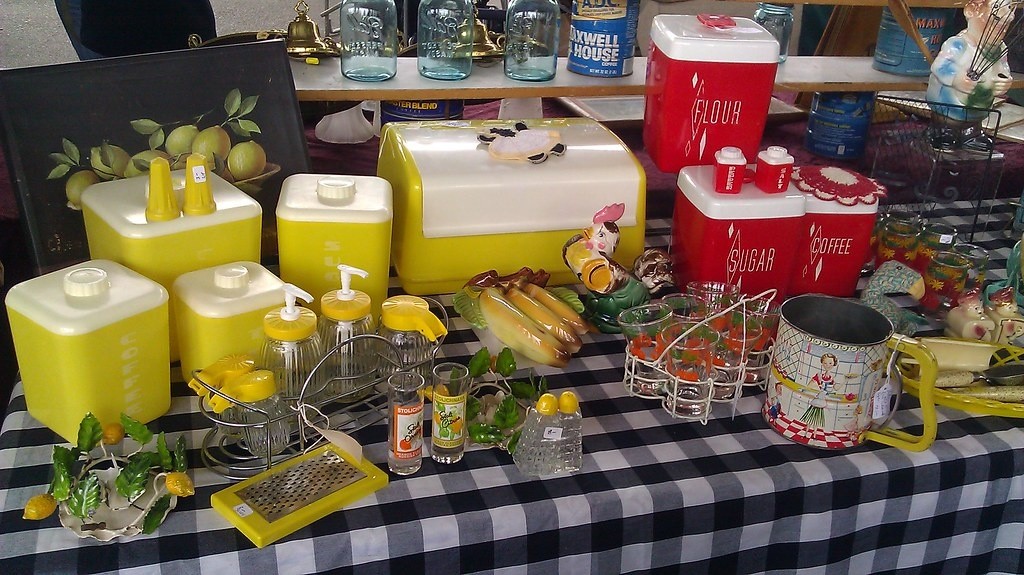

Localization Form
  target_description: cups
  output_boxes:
[878,210,992,319]
[388,372,425,475]
[431,362,469,464]
[762,294,936,453]
[618,279,780,420]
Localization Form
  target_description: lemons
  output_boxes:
[64,124,265,210]
[536,390,577,415]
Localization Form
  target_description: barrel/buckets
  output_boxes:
[567,0,640,78]
[381,100,464,125]
[802,91,877,158]
[640,15,878,306]
[871,7,957,78]
[5,168,393,446]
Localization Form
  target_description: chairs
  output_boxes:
[1,38,314,277]
[54,0,218,62]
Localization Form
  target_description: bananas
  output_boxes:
[480,282,591,368]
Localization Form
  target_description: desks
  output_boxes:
[0,194,1024,574]
[285,48,1023,102]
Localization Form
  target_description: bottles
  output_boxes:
[714,147,747,196]
[145,157,181,223]
[341,0,397,83]
[512,393,563,475]
[189,266,448,458]
[559,391,582,473]
[417,0,473,80]
[756,146,795,194]
[504,0,561,81]
[754,3,794,63]
[181,154,216,217]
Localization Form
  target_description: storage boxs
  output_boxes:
[6,12,889,446]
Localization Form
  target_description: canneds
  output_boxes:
[566,0,641,78]
[802,91,879,161]
[872,5,948,77]
